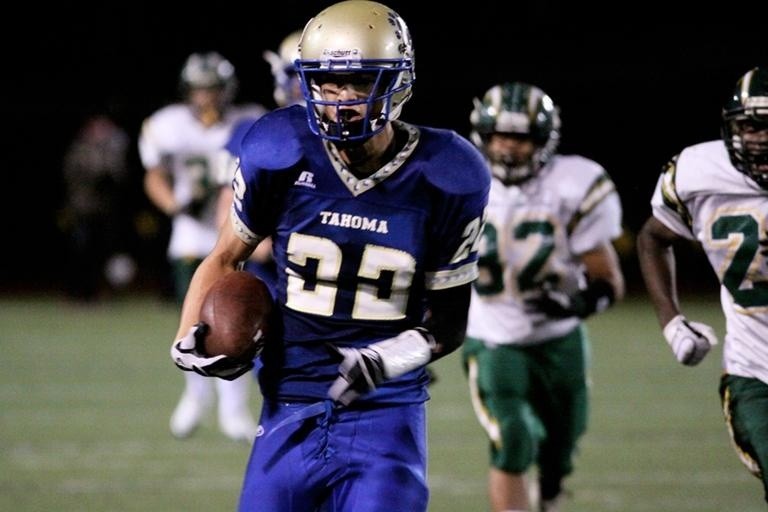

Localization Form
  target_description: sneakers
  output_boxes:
[169,371,257,442]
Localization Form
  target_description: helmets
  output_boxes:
[470,83,560,147]
[722,65,768,135]
[279,0,415,112]
[179,52,237,89]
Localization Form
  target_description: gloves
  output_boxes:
[322,341,386,409]
[171,323,254,381]
[662,314,719,367]
[523,281,576,329]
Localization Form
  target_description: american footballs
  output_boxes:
[199,269,273,356]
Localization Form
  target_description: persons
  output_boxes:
[461,81,629,511]
[634,68,767,512]
[214,29,307,380]
[137,50,258,437]
[168,1,494,511]
